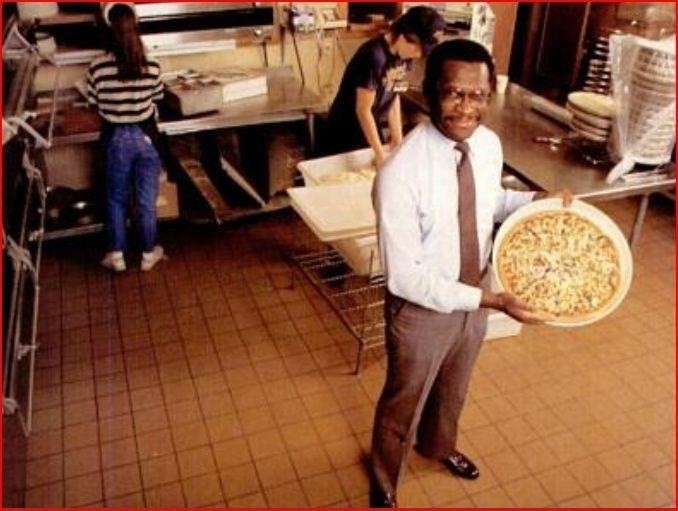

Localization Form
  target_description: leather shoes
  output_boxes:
[440,448,480,480]
[369,478,397,508]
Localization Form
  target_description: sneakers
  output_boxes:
[100,250,126,272]
[140,245,164,271]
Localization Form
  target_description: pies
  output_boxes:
[497,210,622,323]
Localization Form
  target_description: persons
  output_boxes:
[83,1,166,272]
[325,7,446,290]
[369,37,572,509]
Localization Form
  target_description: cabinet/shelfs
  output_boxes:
[291,185,391,378]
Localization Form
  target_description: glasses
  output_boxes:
[436,85,489,104]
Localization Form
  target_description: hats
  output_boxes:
[388,5,459,34]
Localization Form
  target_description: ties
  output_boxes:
[454,141,481,287]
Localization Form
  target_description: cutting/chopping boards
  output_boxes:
[489,194,634,330]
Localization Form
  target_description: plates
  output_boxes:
[567,89,615,143]
[619,37,677,165]
